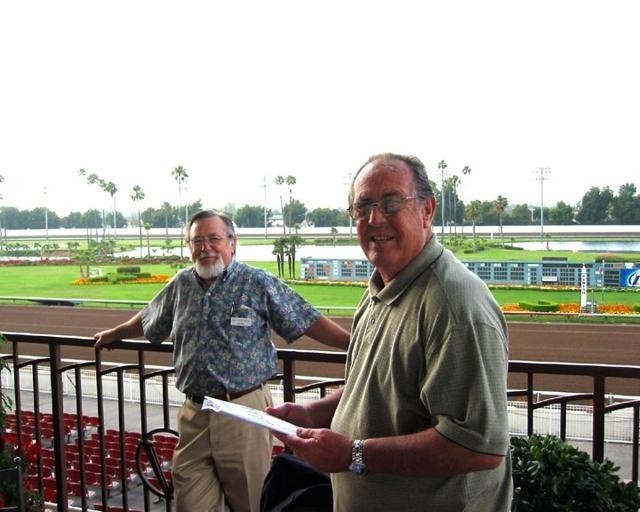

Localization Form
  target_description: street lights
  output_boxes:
[535,167,550,242]
[342,170,359,237]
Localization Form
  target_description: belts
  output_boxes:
[186,383,265,404]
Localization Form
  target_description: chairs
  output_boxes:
[0,409,286,511]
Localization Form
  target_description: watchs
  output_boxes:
[349,439,367,478]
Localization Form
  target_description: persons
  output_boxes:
[266,153,516,511]
[94,210,351,512]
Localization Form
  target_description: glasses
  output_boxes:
[346,195,417,219]
